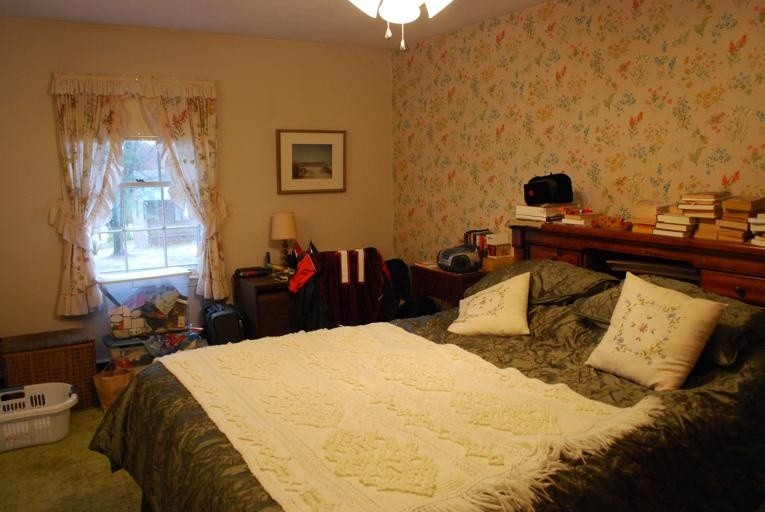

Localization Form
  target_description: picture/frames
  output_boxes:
[276,128,347,195]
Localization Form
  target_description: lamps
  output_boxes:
[348,0,456,26]
[270,212,295,263]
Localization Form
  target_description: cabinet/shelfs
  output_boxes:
[234,275,299,340]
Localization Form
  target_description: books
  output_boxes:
[464,229,510,259]
[509,205,600,227]
[632,192,765,246]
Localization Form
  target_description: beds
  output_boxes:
[87,220,765,512]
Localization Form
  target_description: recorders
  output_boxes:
[437,244,481,272]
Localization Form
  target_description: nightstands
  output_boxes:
[411,260,488,318]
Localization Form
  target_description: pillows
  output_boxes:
[567,274,765,366]
[465,258,620,308]
[448,272,532,338]
[585,270,731,394]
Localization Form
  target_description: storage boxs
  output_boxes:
[93,264,200,368]
